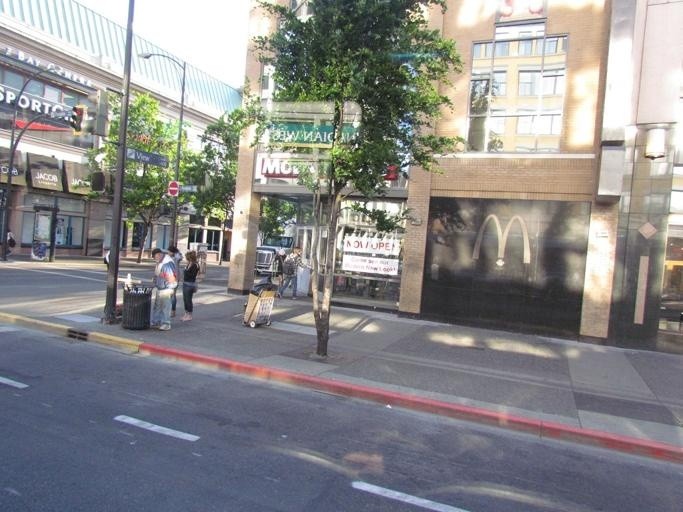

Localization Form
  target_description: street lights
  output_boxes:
[250,283,276,296]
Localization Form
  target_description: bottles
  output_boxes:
[151,249,160,258]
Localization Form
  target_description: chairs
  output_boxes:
[158,323,170,331]
[179,316,192,321]
[149,320,160,326]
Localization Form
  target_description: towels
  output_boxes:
[32,240,47,261]
[122,288,152,330]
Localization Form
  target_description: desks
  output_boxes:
[0,68,66,262]
[136,52,187,251]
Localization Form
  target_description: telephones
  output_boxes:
[126,147,169,169]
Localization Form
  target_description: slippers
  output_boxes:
[282,254,299,275]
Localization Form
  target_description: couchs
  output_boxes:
[71,106,84,133]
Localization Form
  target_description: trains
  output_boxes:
[166,181,180,197]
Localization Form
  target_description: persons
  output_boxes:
[273,249,287,288]
[277,247,312,299]
[180,250,200,321]
[4,228,15,258]
[103,246,110,270]
[55,217,65,246]
[148,248,178,331]
[166,245,182,318]
